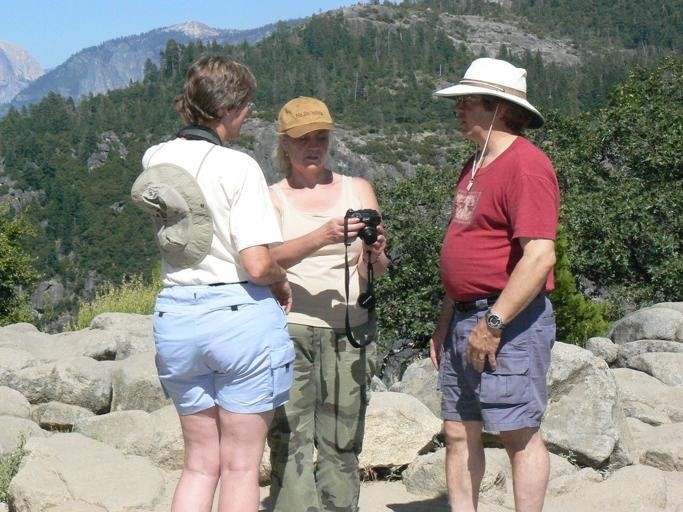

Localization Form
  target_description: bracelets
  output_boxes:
[362,256,379,265]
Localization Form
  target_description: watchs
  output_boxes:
[485,307,506,331]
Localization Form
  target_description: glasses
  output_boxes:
[455,95,498,105]
[239,103,256,111]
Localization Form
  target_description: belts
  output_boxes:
[454,298,496,313]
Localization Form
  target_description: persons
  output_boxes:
[267,96,388,512]
[142,55,296,512]
[429,57,560,512]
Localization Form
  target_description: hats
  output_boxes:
[433,58,545,128]
[129,163,215,269]
[277,96,336,141]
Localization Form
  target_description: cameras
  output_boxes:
[348,209,381,245]
